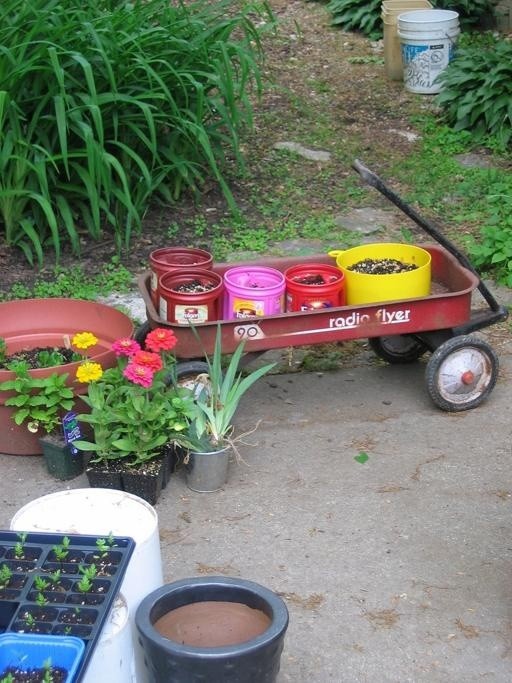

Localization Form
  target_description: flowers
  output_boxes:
[71,358,119,466]
[129,347,167,385]
[113,361,172,468]
[68,330,108,363]
[106,335,143,393]
[141,325,178,374]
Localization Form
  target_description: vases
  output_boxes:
[83,461,123,491]
[126,463,164,506]
[0,298,136,458]
[130,570,289,683]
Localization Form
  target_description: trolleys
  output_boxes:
[134,158,510,413]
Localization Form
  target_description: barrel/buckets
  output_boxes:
[223,267,286,320]
[149,247,213,314]
[397,10,460,94]
[159,269,222,324]
[379,2,433,78]
[328,243,431,305]
[284,264,344,312]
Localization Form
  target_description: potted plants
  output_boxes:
[172,318,280,496]
[1,358,85,482]
